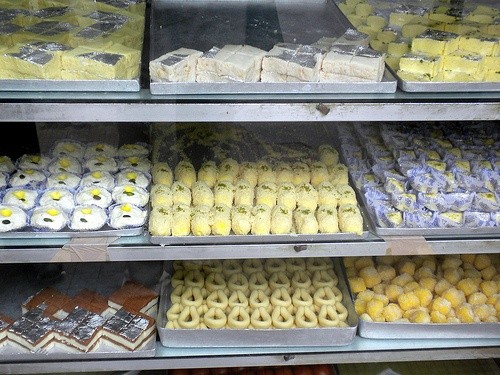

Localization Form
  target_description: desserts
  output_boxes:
[1,0,500,353]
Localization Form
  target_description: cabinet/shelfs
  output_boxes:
[1,89,500,375]
